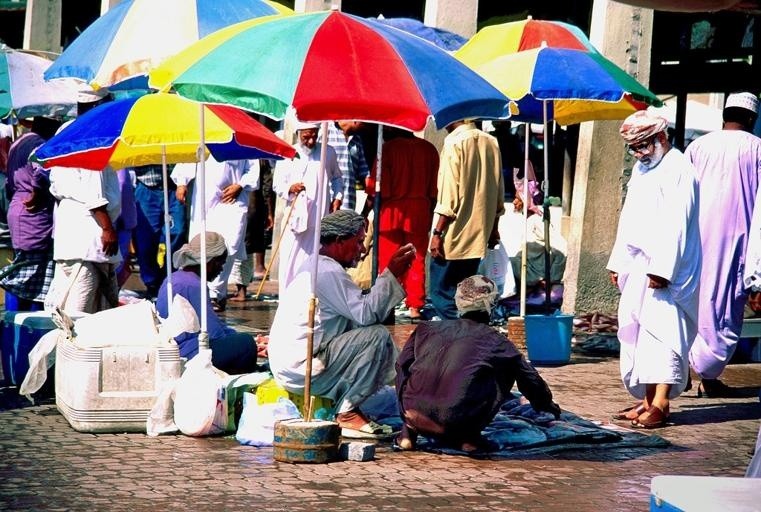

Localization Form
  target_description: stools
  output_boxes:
[287,392,332,419]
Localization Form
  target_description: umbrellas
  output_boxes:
[25,93,298,317]
[147,7,517,420]
[452,17,601,317]
[0,44,108,143]
[474,41,665,317]
[44,1,298,93]
[364,17,472,287]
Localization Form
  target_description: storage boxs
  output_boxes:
[54,335,186,432]
[0,309,92,395]
[643,469,761,511]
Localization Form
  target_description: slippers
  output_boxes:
[342,420,401,440]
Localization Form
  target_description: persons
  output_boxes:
[606,109,703,428]
[1,118,507,325]
[156,230,257,374]
[685,91,761,394]
[392,275,562,453]
[743,181,761,477]
[269,210,417,436]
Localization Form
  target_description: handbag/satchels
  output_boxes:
[348,220,373,291]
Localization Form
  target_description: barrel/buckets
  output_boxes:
[523,314,576,365]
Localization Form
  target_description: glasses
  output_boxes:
[627,142,653,156]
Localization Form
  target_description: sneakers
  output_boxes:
[697,378,733,397]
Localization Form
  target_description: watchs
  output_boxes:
[433,229,445,237]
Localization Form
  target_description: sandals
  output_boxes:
[613,399,670,428]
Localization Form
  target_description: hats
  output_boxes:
[725,91,758,114]
